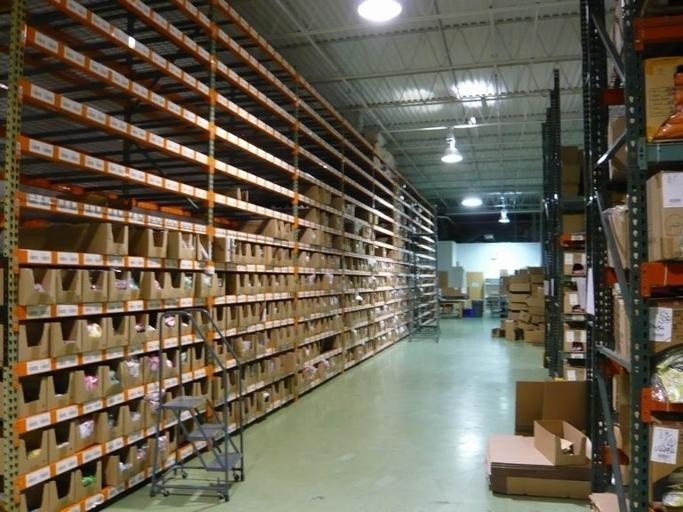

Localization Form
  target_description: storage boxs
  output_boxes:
[480,51,683,512]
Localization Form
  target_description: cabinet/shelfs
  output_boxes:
[297,76,438,400]
[0,1,301,511]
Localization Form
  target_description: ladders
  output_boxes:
[410,232,440,342]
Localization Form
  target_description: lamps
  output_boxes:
[459,182,484,209]
[441,124,463,163]
